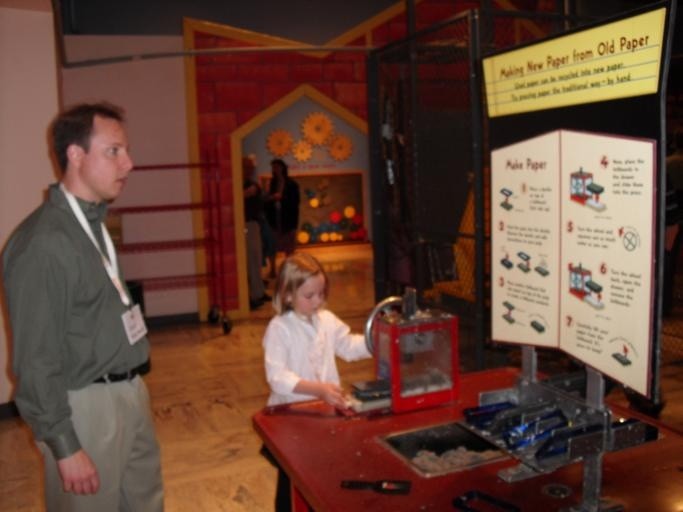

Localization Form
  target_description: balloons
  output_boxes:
[331,223,339,231]
[306,191,314,199]
[344,206,356,218]
[359,229,367,239]
[342,231,349,241]
[353,213,363,224]
[343,221,349,228]
[350,231,358,240]
[297,231,309,243]
[331,233,339,242]
[331,211,343,223]
[303,221,312,230]
[348,220,354,227]
[320,232,329,242]
[310,198,319,207]
[320,223,330,232]
[311,235,317,242]
[338,235,344,241]
[313,227,320,235]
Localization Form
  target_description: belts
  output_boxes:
[93,368,138,384]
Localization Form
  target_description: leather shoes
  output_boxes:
[252,300,264,307]
[262,295,272,300]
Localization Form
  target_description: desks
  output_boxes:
[249,366,681,512]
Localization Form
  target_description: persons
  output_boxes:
[253,190,277,286]
[2,104,165,512]
[242,154,272,311]
[369,97,425,227]
[259,254,372,511]
[263,160,299,255]
